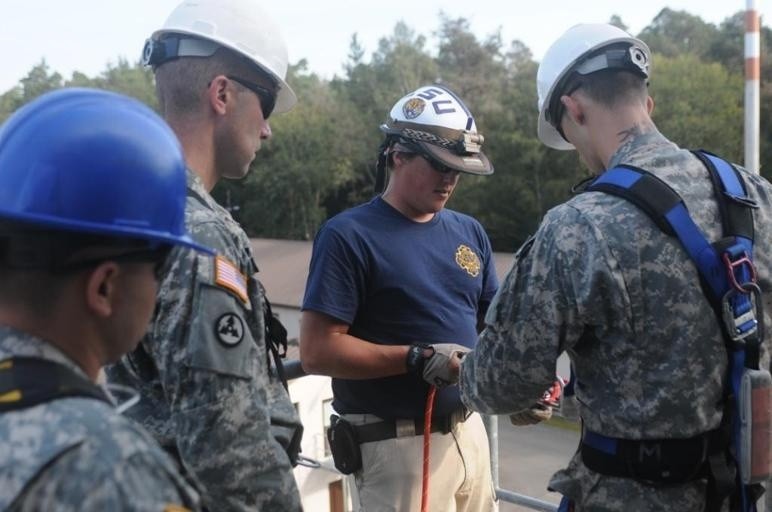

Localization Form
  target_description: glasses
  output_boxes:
[208,74,274,119]
[72,245,184,279]
[400,141,451,172]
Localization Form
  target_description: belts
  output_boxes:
[353,406,472,443]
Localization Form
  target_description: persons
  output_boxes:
[458,18,772,511]
[299,85,557,512]
[100,2,301,512]
[0,87,209,511]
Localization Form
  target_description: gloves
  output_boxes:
[511,405,552,426]
[421,343,473,389]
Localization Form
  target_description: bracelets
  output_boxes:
[405,343,422,374]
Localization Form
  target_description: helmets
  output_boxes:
[0,88,218,259]
[151,0,296,112]
[536,22,651,151]
[381,85,494,175]
